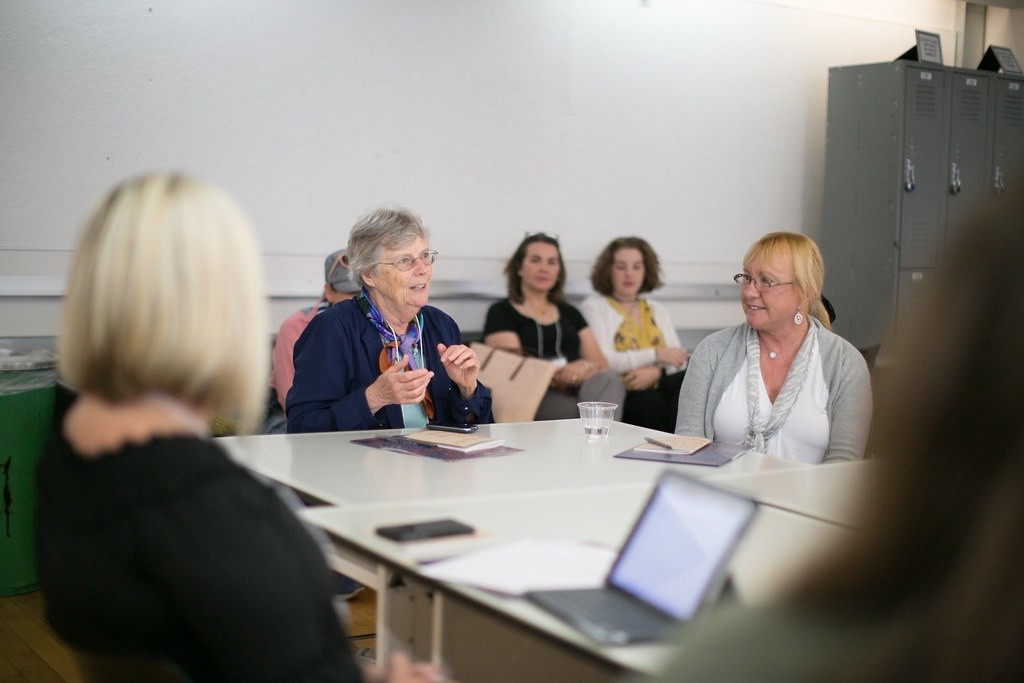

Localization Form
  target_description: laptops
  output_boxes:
[524,469,761,646]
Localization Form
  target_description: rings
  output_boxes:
[572,374,578,380]
[473,352,477,358]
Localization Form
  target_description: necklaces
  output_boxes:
[525,302,552,317]
[755,333,807,358]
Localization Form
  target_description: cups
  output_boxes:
[577,402,618,440]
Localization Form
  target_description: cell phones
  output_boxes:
[375,518,475,543]
[427,422,478,434]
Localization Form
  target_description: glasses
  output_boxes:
[525,231,560,242]
[734,273,798,291]
[377,249,439,271]
[327,253,349,280]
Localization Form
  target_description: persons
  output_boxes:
[33,170,449,683]
[257,208,689,602]
[674,232,873,469]
[653,182,1024,682]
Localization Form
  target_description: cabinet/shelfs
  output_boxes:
[820,60,1024,351]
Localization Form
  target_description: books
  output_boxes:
[633,434,712,456]
[401,430,502,453]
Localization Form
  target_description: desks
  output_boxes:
[214,419,874,683]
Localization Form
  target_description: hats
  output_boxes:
[325,250,363,292]
